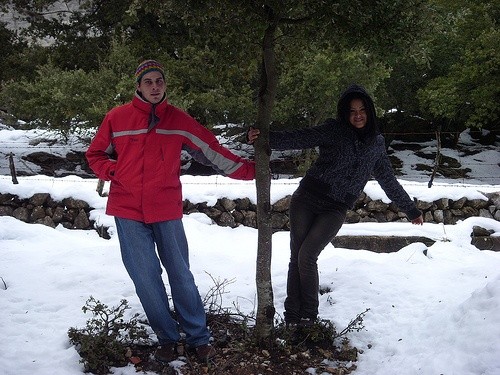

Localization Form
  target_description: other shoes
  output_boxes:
[284,310,314,329]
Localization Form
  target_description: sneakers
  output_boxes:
[155,343,178,363]
[188,344,215,364]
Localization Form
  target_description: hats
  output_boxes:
[135,58,166,83]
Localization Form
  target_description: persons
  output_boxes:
[85,61,273,365]
[248,86,424,325]
